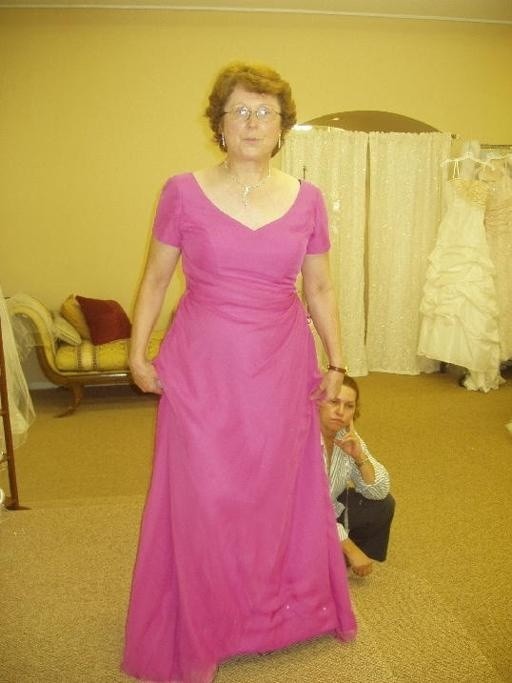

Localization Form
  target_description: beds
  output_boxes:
[7,294,166,418]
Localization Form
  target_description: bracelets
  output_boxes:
[354,458,370,465]
[327,365,346,374]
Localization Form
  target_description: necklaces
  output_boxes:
[220,158,272,215]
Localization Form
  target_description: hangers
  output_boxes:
[440,152,493,170]
[482,148,512,171]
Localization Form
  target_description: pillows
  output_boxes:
[75,295,132,346]
[52,310,81,346]
[60,294,90,339]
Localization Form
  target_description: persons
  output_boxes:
[319,374,395,577]
[120,65,358,683]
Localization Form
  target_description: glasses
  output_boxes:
[219,104,283,122]
